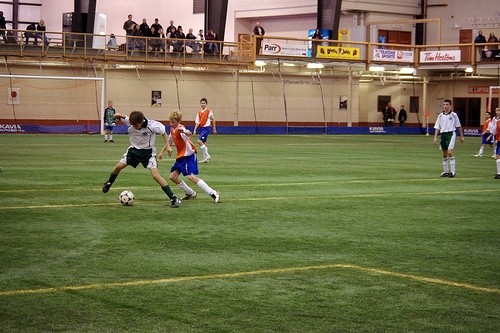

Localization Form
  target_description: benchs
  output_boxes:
[0,36,83,47]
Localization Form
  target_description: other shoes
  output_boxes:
[495,175,500,179]
[473,154,482,158]
[204,153,211,159]
[449,172,456,178]
[491,155,497,159]
[109,140,114,143]
[198,159,208,163]
[441,173,449,177]
[104,140,107,142]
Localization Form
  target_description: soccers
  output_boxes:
[119,190,135,206]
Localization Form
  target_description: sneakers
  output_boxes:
[102,181,112,193]
[181,191,197,200]
[210,190,220,203]
[170,197,182,207]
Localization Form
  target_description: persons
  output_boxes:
[433,99,465,177]
[474,30,500,62]
[103,100,116,143]
[312,28,322,57]
[107,34,117,50]
[382,101,397,127]
[474,107,500,179]
[24,20,49,49]
[398,105,407,127]
[0,10,8,43]
[102,111,182,207]
[379,34,386,49]
[157,112,220,203]
[253,22,265,56]
[193,98,218,163]
[152,92,160,106]
[123,14,219,56]
